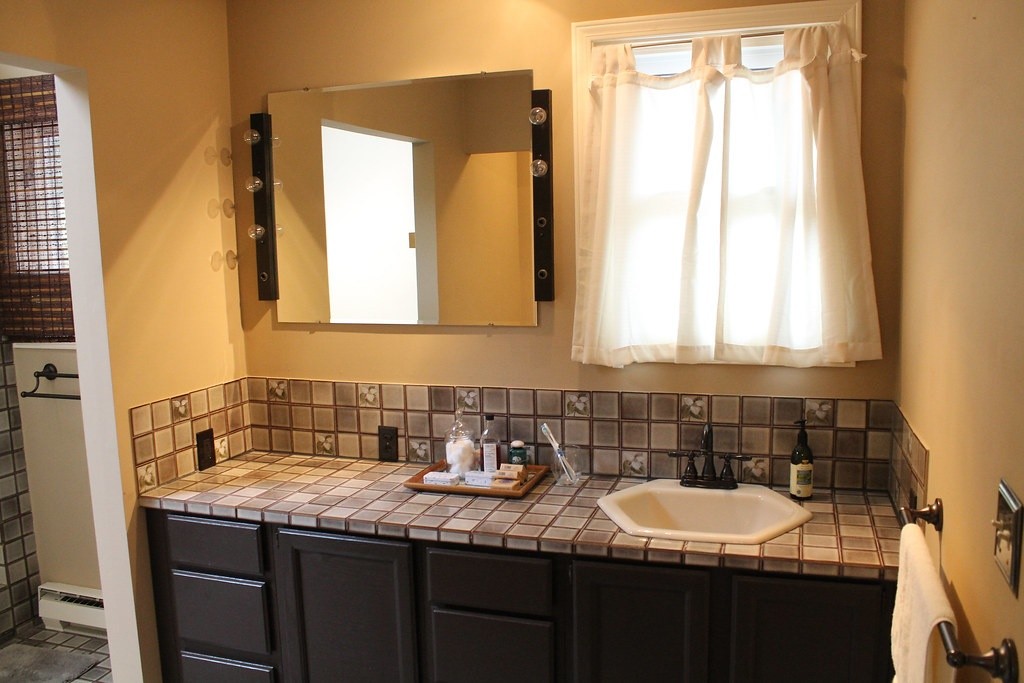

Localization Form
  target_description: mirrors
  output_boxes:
[262,70,540,330]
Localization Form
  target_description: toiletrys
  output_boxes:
[790,418,814,499]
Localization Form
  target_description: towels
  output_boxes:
[890,525,957,682]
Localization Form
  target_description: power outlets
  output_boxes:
[908,488,918,522]
[378,426,399,463]
[196,427,216,471]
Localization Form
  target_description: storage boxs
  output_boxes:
[495,469,524,483]
[490,479,521,492]
[500,464,528,481]
[423,471,459,485]
[464,470,495,486]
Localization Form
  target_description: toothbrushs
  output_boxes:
[541,422,577,483]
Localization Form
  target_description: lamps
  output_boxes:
[242,106,279,306]
[527,86,553,304]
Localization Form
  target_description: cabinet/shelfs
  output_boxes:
[565,559,893,683]
[271,527,564,683]
[145,505,284,683]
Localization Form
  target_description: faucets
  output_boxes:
[701,422,716,479]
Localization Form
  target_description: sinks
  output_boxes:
[596,478,814,545]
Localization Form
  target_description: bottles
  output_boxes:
[479,414,501,472]
[507,440,527,469]
[443,410,475,481]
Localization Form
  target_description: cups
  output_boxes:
[550,444,583,487]
